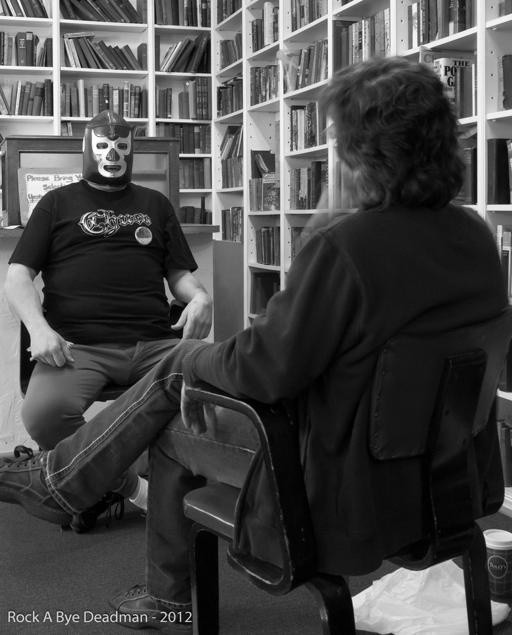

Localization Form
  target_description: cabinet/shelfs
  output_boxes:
[3,2,512,466]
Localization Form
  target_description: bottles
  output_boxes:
[483,529,512,596]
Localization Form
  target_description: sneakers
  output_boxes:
[73,492,122,532]
[0,445,73,527]
[107,583,193,634]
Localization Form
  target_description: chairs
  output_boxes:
[167,304,509,634]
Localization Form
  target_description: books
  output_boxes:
[0,0,511,490]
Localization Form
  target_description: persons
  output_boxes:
[1,53,510,635]
[3,109,214,533]
